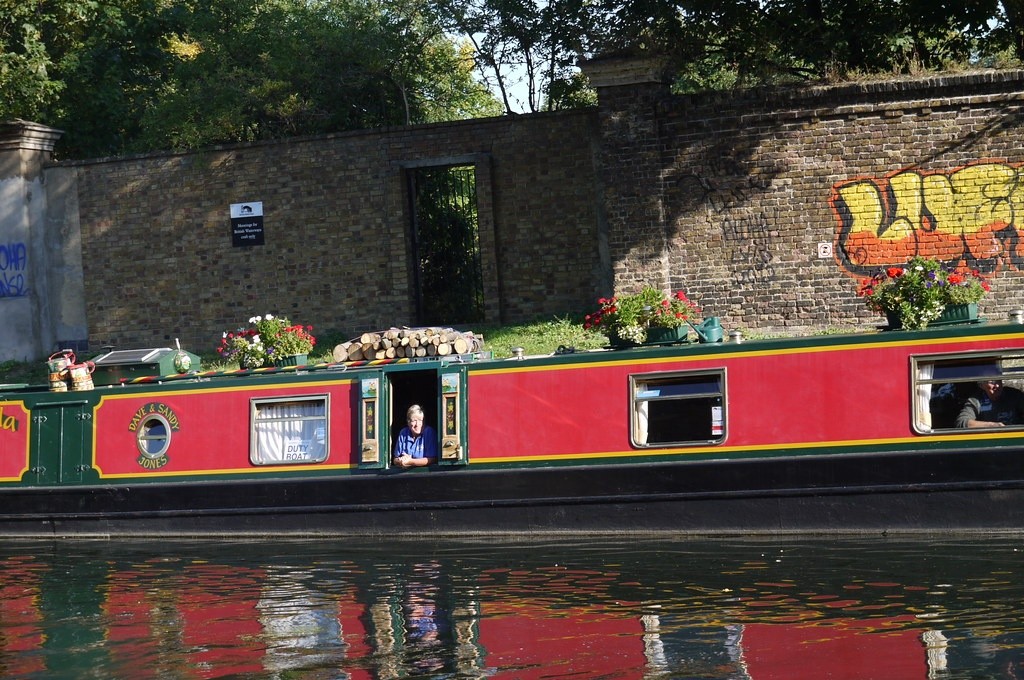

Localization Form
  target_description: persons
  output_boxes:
[394,404,438,467]
[930,362,1024,428]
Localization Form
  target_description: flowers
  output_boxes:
[580,286,701,345]
[217,313,316,367]
[859,255,989,330]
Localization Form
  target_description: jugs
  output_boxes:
[687,317,723,343]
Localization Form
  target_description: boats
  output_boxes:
[0,324,1024,550]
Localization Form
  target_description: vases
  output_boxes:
[240,352,308,370]
[610,323,690,348]
[887,303,977,327]
